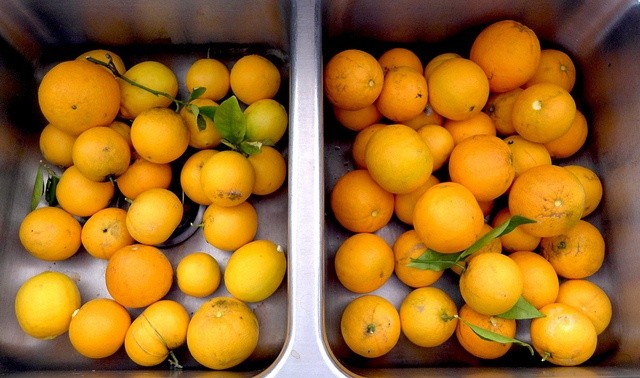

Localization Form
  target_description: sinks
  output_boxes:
[307,2,640,378]
[0,0,305,378]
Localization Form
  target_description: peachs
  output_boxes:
[180,151,220,206]
